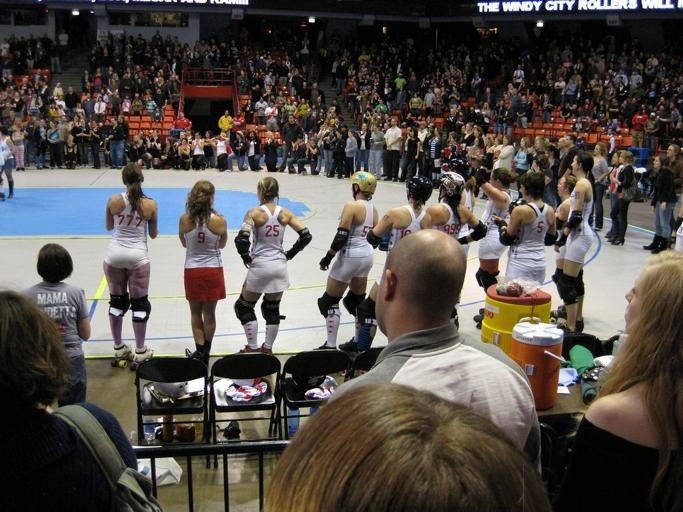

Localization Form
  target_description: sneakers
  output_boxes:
[381,173,403,182]
[239,343,272,354]
[593,226,624,246]
[323,170,350,179]
[15,166,25,172]
[37,164,64,170]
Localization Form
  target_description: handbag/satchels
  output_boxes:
[54,404,163,512]
[622,179,639,201]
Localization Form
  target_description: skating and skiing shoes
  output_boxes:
[313,338,366,357]
[474,308,485,330]
[550,304,585,333]
[111,343,154,371]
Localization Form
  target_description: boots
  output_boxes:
[644,235,668,254]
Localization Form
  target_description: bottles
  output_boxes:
[191,415,204,433]
[287,403,301,438]
[142,412,156,441]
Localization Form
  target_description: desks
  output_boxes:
[535,381,593,418]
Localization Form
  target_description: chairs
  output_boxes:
[238,93,251,108]
[353,346,385,378]
[245,123,285,153]
[133,355,210,469]
[272,351,352,439]
[211,352,281,469]
[505,94,633,151]
[434,111,450,131]
[393,109,401,126]
[14,67,50,89]
[461,96,477,110]
[81,92,176,144]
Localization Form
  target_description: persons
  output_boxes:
[23,244,92,403]
[355,175,432,357]
[0,290,140,512]
[554,150,595,332]
[490,172,558,295]
[556,251,683,512]
[327,228,542,477]
[262,382,554,512]
[474,166,520,329]
[426,172,486,327]
[179,180,227,365]
[235,176,311,356]
[1,30,683,254]
[104,164,157,363]
[317,170,380,351]
[550,174,576,318]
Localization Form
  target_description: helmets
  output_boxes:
[405,175,433,202]
[439,172,466,197]
[153,380,189,397]
[293,372,325,390]
[350,171,377,194]
